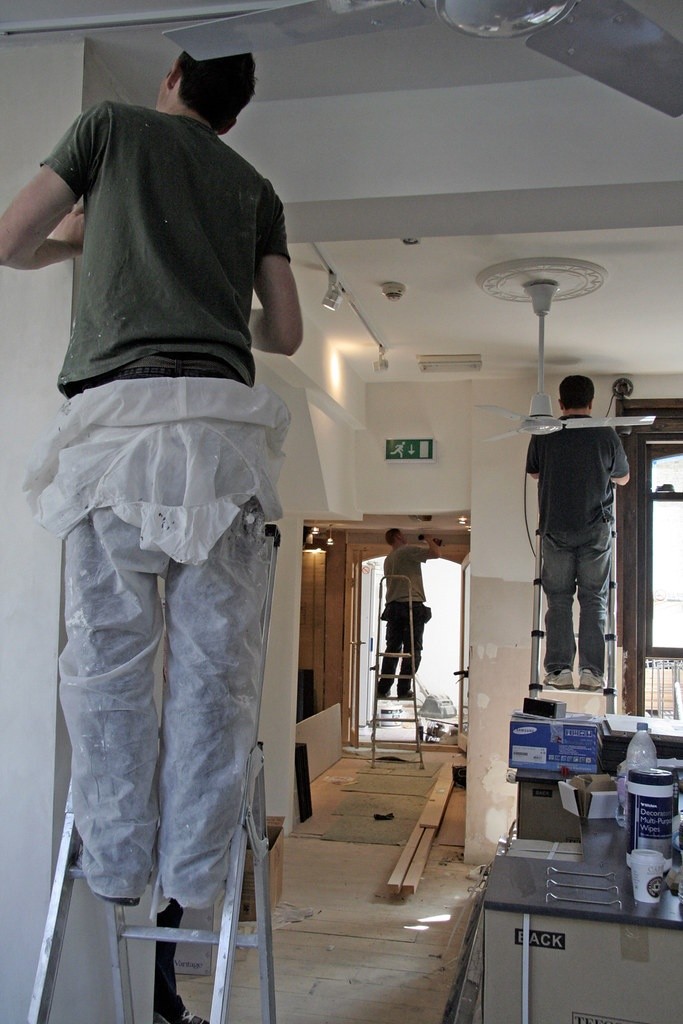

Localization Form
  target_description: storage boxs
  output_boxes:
[509,709,598,773]
[239,815,283,922]
[508,838,581,862]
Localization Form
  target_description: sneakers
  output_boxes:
[579,669,605,691]
[153,995,208,1024]
[544,667,575,689]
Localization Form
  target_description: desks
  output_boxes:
[482,769,683,1024]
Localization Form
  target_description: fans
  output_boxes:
[159,0,683,119]
[473,283,657,443]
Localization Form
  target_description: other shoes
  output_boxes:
[375,690,392,698]
[398,689,415,699]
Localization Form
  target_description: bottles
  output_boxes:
[626,767,674,872]
[624,722,657,831]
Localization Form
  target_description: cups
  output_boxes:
[629,849,664,904]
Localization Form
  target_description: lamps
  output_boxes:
[372,346,388,373]
[321,274,344,311]
[326,525,335,545]
[311,520,320,535]
[417,355,482,372]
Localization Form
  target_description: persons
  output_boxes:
[377,528,440,700]
[526,375,631,691]
[153,897,210,1024]
[1,50,303,909]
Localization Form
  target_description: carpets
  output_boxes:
[318,745,444,846]
